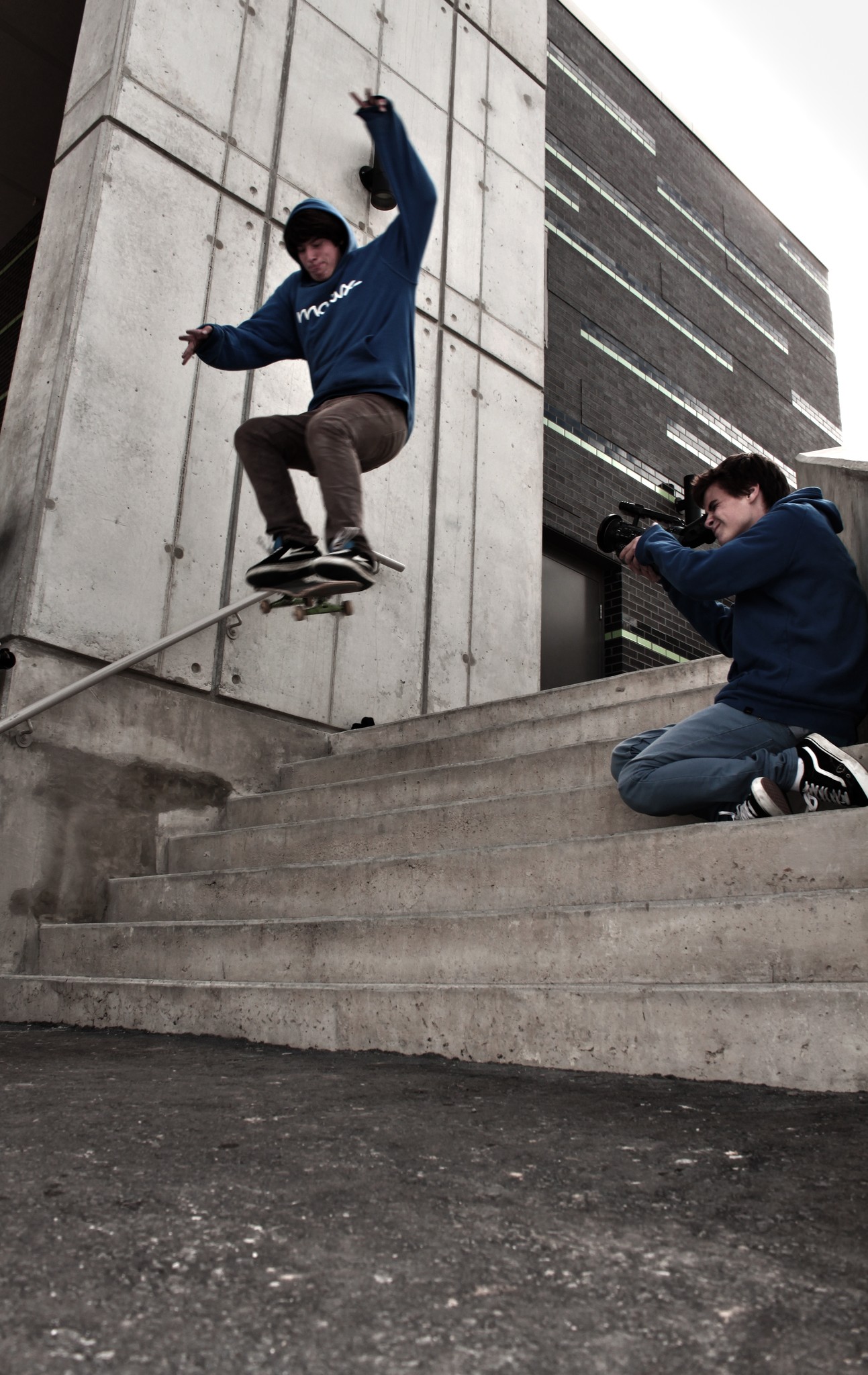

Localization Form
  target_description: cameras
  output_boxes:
[596,500,717,575]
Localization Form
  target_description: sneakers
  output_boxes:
[244,538,323,588]
[312,542,380,590]
[728,777,790,823]
[795,732,868,808]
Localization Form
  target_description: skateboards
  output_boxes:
[258,580,361,621]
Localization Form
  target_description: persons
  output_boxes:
[187,88,438,581]
[609,450,868,819]
